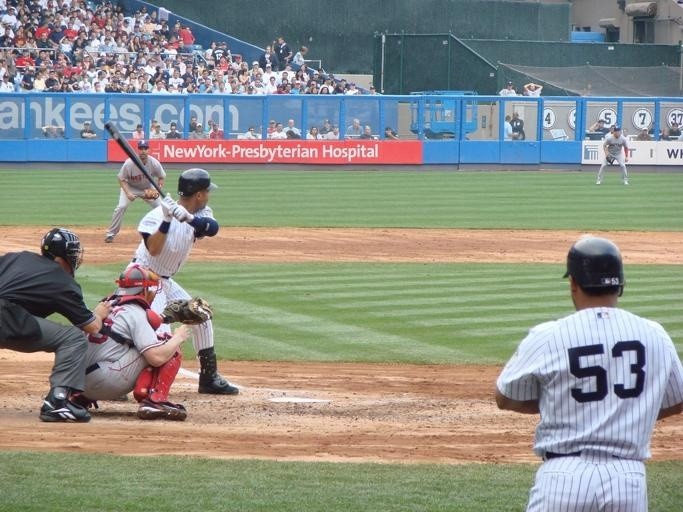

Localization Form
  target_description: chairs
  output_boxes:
[548,128,569,141]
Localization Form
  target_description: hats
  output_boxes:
[196,123,203,127]
[614,126,621,131]
[137,140,149,148]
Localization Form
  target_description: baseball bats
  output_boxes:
[103,119,165,197]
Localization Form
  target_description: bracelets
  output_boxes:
[159,221,170,234]
[188,218,201,228]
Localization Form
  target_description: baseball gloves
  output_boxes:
[145,188,160,200]
[606,153,618,167]
[161,297,213,323]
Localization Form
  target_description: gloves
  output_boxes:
[159,192,194,224]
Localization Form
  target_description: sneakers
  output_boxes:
[137,400,187,421]
[38,396,92,424]
[196,376,241,395]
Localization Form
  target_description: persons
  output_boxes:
[106,141,167,244]
[0,226,116,423]
[129,167,238,395]
[590,118,681,187]
[492,235,682,512]
[500,81,543,140]
[69,261,194,421]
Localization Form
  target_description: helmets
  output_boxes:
[563,234,625,290]
[112,266,163,297]
[177,168,218,195]
[41,228,83,271]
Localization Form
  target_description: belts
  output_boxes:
[541,451,582,460]
